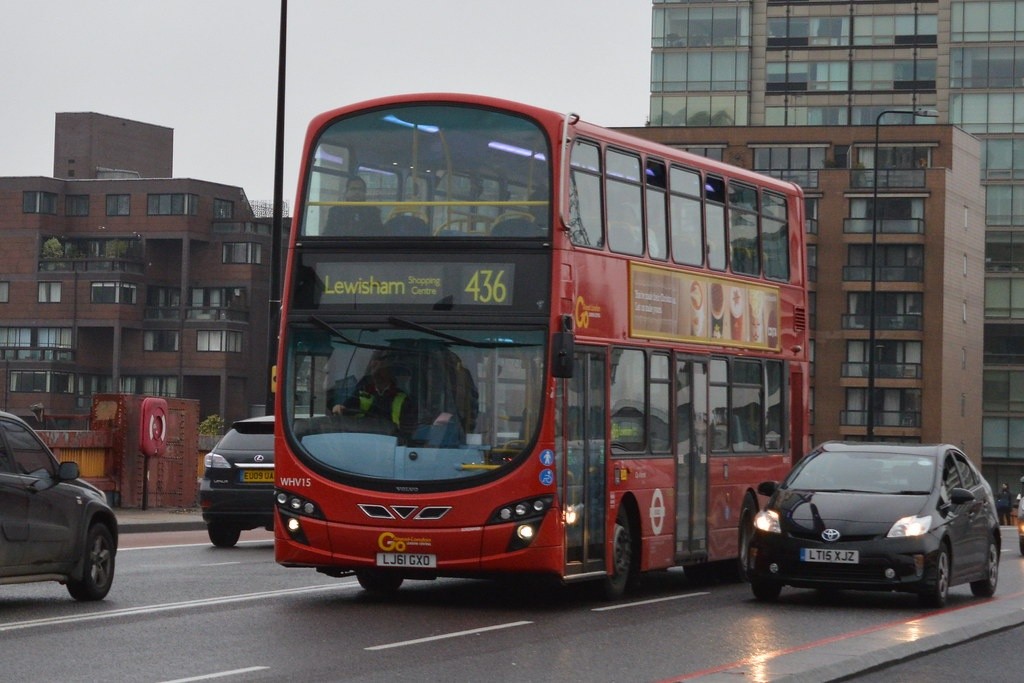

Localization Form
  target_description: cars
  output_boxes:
[198,411,330,548]
[744,436,1006,611]
[1014,477,1024,553]
[0,410,120,603]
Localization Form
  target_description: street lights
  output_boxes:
[866,109,941,439]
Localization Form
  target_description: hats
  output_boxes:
[373,368,398,385]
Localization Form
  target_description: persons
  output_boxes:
[327,177,385,233]
[998,484,1012,525]
[330,363,416,440]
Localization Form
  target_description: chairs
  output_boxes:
[382,206,550,236]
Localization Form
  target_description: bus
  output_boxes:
[265,90,816,606]
